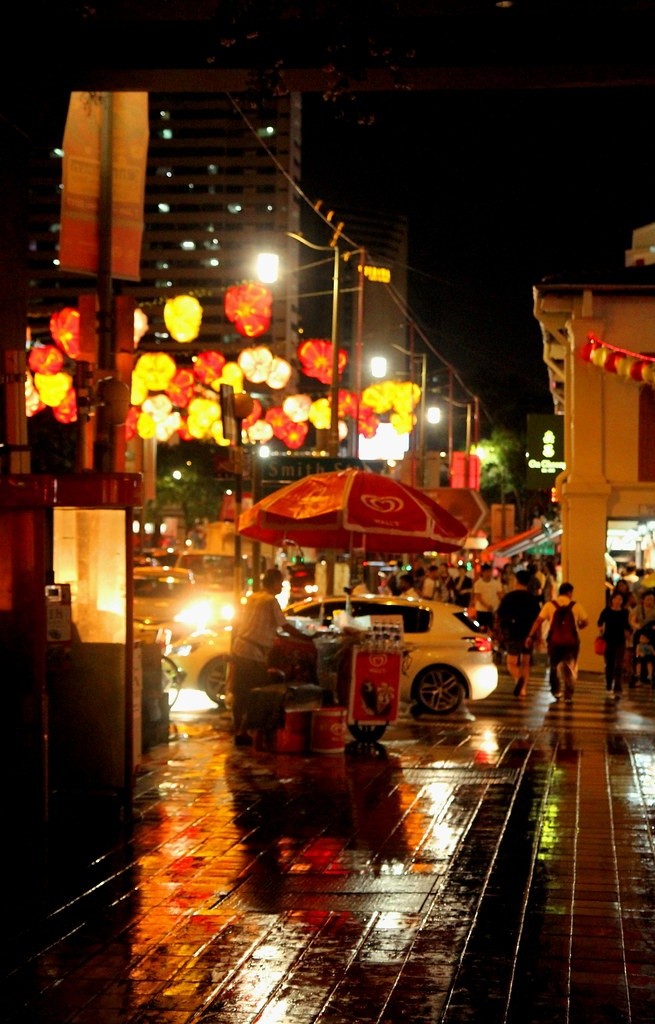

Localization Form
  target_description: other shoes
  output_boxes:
[234,732,253,747]
[633,673,641,679]
[605,689,616,699]
[645,677,652,682]
[554,691,564,697]
[513,676,524,697]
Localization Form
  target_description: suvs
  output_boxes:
[161,591,497,717]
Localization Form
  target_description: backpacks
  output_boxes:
[546,598,581,660]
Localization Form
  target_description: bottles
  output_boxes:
[364,621,401,650]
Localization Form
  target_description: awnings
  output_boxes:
[484,518,562,559]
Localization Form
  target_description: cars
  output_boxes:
[131,546,249,647]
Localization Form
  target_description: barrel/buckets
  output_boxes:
[310,707,348,753]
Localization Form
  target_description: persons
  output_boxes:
[228,569,315,745]
[598,549,655,694]
[349,550,559,640]
[525,582,589,703]
[498,570,540,696]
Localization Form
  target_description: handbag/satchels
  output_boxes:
[593,633,607,655]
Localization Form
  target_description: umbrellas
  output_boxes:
[237,466,469,550]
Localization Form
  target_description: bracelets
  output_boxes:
[528,635,532,638]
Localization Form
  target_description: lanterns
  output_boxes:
[21,285,422,444]
[580,339,655,386]
